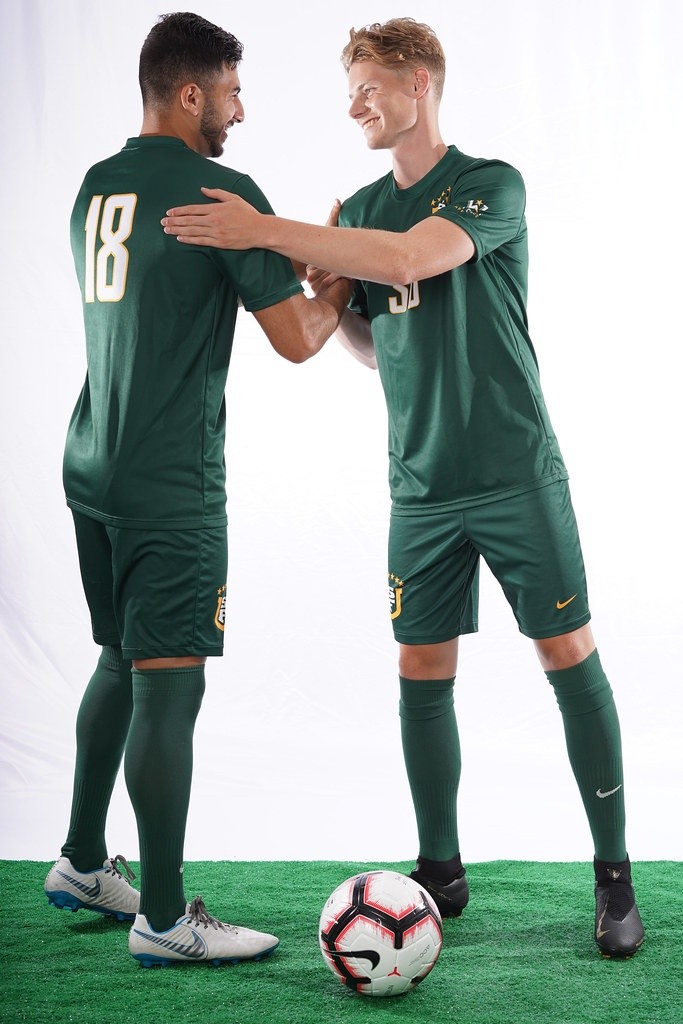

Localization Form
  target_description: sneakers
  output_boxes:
[593,880,644,958]
[44,855,140,921]
[128,895,279,968]
[407,870,469,917]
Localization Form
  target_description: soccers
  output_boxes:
[317,868,443,997]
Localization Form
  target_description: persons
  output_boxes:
[160,16,651,961]
[39,12,354,966]
